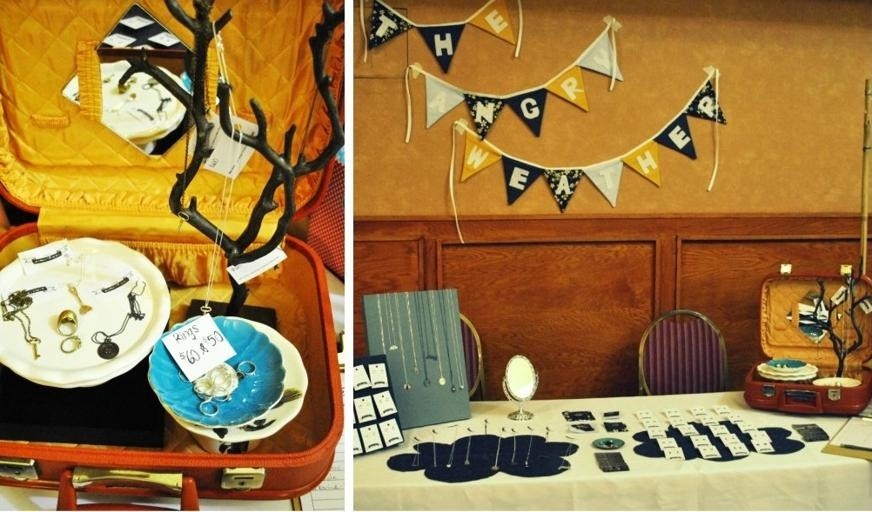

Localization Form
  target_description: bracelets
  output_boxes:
[792,301,799,329]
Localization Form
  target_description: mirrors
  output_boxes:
[501,354,539,421]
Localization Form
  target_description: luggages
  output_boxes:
[0,0,344,511]
[742,270,872,417]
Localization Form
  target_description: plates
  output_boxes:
[756,359,818,382]
[148,315,287,429]
[814,376,860,390]
[0,235,170,388]
[158,314,309,444]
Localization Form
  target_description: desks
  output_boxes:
[354,391,872,511]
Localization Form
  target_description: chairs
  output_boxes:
[459,312,487,401]
[638,309,730,395]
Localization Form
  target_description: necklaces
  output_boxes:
[76,41,181,139]
[376,288,468,393]
[843,287,851,374]
[199,21,241,317]
[176,29,201,231]
[90,280,146,360]
[407,421,574,472]
[0,290,48,359]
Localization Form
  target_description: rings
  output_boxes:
[188,361,258,416]
[62,338,84,353]
[57,309,78,336]
